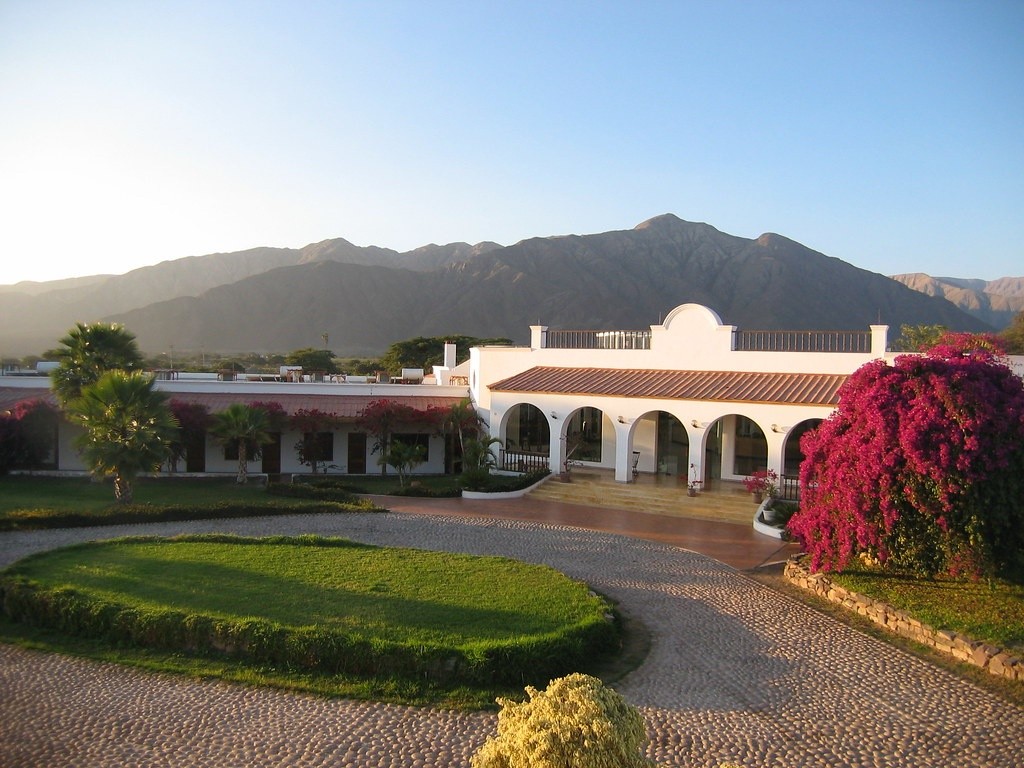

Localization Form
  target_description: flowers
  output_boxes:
[686,463,704,489]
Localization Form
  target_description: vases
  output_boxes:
[687,489,696,497]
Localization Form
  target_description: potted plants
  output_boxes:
[762,483,781,521]
[561,458,584,483]
[744,469,779,503]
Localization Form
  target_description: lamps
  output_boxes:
[691,420,697,428]
[771,424,777,432]
[617,416,624,423]
[550,411,557,419]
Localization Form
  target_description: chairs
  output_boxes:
[632,451,640,476]
